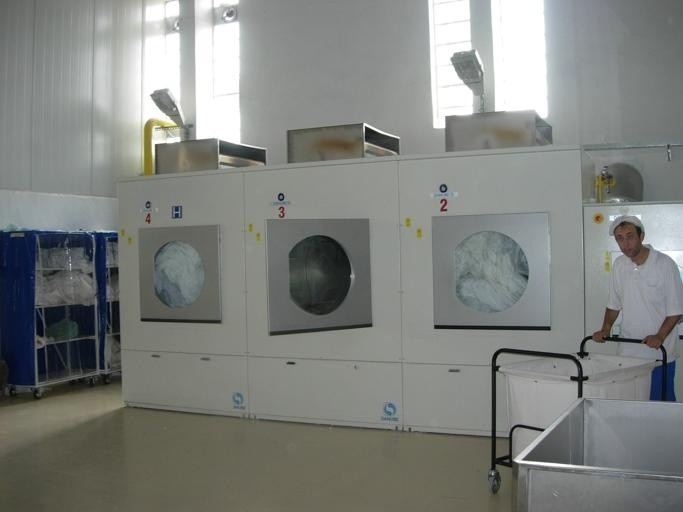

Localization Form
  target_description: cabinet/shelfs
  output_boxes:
[94,231,118,385]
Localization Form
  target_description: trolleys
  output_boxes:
[487,336,668,496]
[68,230,122,387]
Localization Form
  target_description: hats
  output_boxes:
[608,216,644,235]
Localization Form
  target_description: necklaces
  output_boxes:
[633,247,647,271]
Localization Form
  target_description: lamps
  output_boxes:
[150,89,190,143]
[451,50,501,117]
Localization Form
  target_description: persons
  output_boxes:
[592,214,683,402]
